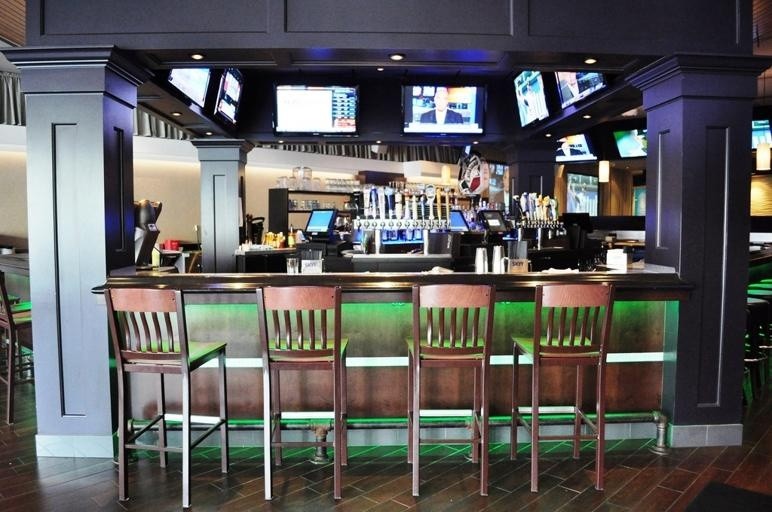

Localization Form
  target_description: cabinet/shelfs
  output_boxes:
[269,188,354,248]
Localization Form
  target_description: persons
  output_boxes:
[556,136,584,158]
[420,85,463,124]
[559,70,594,101]
[630,129,649,157]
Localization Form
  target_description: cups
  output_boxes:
[492,243,506,273]
[274,166,362,191]
[474,244,489,274]
[287,257,299,275]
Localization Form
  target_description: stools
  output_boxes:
[744,279,772,405]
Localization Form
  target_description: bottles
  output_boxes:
[264,224,295,248]
[520,192,560,223]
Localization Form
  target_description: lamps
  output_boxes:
[756,70,771,172]
[598,121,610,183]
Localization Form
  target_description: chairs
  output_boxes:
[405,281,500,497]
[0,295,23,370]
[509,281,618,493]
[103,284,229,509]
[0,270,35,425]
[255,284,350,500]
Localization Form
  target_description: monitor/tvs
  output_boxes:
[552,132,598,164]
[562,212,593,234]
[165,68,211,107]
[512,70,553,130]
[214,66,251,130]
[476,209,509,234]
[236,255,288,273]
[611,128,650,160]
[273,83,360,137]
[304,208,337,234]
[135,201,163,268]
[483,161,512,215]
[567,171,600,216]
[528,247,577,271]
[448,208,471,234]
[400,82,488,136]
[552,72,611,109]
